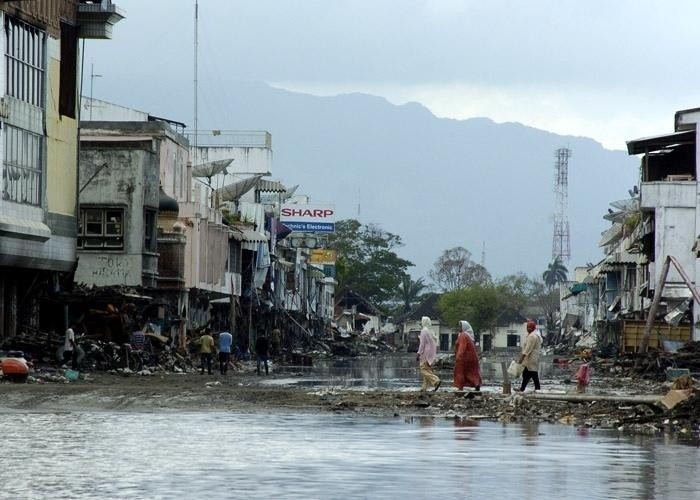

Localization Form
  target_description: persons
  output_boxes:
[451,320,482,391]
[217,324,233,374]
[131,325,145,371]
[57,321,79,370]
[197,327,214,375]
[254,327,270,376]
[414,315,442,391]
[512,320,544,391]
[270,324,283,355]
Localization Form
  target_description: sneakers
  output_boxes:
[513,387,542,392]
[420,380,482,391]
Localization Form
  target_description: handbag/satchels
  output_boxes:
[507,360,527,378]
